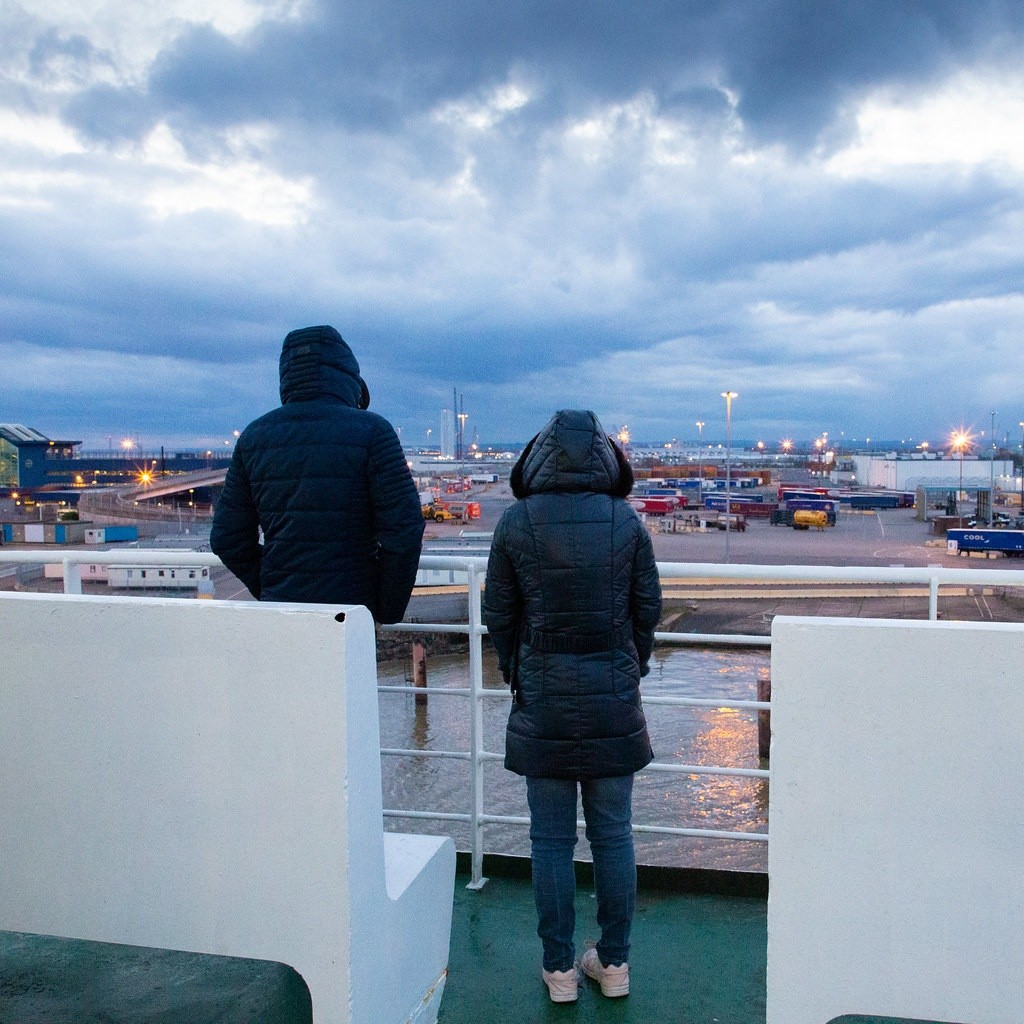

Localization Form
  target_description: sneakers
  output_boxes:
[582,949,629,997]
[542,968,579,1001]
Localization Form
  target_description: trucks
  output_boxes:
[433,501,480,519]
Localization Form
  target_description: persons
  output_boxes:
[210,324,427,630]
[483,410,664,1000]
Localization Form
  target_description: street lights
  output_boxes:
[428,429,432,487]
[696,421,705,503]
[456,414,469,502]
[988,410,998,528]
[720,391,739,563]
[621,433,627,455]
[955,435,967,527]
[816,440,822,487]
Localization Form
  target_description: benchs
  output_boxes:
[0,593,458,1024]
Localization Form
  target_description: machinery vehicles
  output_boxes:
[421,505,456,523]
